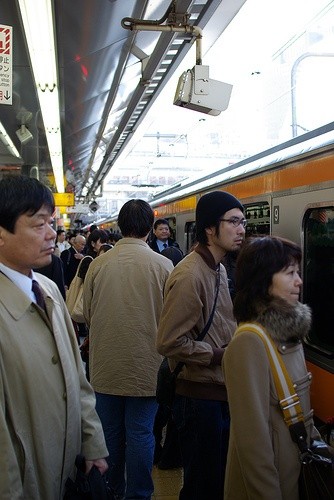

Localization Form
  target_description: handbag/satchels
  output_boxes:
[298,439,334,500]
[62,455,116,500]
[152,358,191,470]
[66,255,94,323]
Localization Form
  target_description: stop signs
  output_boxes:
[0,25,12,104]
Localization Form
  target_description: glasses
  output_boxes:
[219,219,248,227]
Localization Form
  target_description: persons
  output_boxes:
[83,198,175,500]
[158,191,247,500]
[0,174,110,500]
[33,221,194,327]
[223,238,319,500]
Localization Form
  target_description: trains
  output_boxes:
[80,121,334,447]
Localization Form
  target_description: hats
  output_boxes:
[196,191,244,226]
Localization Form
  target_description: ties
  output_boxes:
[32,280,48,317]
[163,244,168,249]
